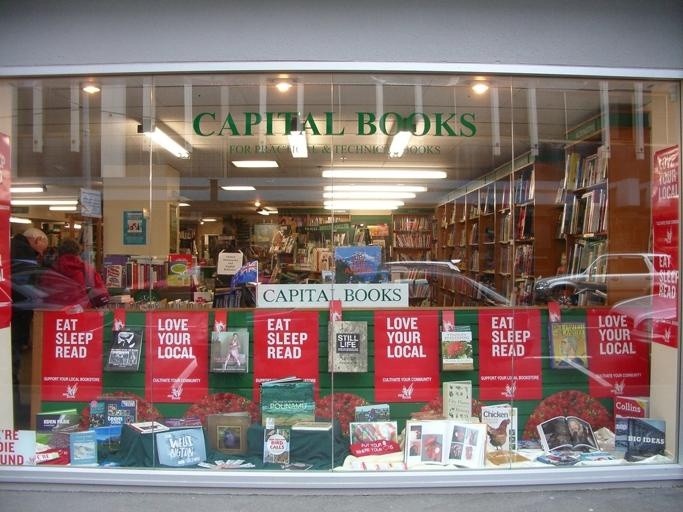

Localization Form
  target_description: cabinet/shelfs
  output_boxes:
[0,101,683,306]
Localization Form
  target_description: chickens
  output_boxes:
[485,417,511,453]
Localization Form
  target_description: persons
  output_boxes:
[38,238,110,309]
[223,333,240,368]
[10,228,48,421]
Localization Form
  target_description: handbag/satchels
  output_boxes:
[81,269,109,309]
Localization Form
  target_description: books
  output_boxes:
[440,325,474,371]
[396,146,608,308]
[327,320,367,373]
[548,320,588,369]
[207,330,249,373]
[350,381,668,471]
[35,375,333,473]
[104,231,242,308]
[103,330,143,372]
[271,217,390,282]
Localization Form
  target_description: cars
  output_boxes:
[0,259,93,351]
[537,253,678,361]
[323,259,513,307]
